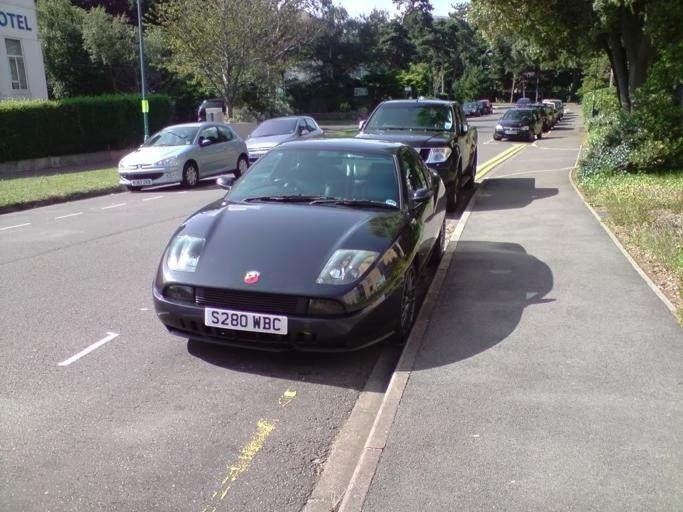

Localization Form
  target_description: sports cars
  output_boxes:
[152,138,448,357]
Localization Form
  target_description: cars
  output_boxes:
[463,97,565,143]
[116,120,250,189]
[243,113,322,165]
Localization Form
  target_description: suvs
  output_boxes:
[353,98,479,210]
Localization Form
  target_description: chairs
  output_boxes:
[355,164,395,203]
